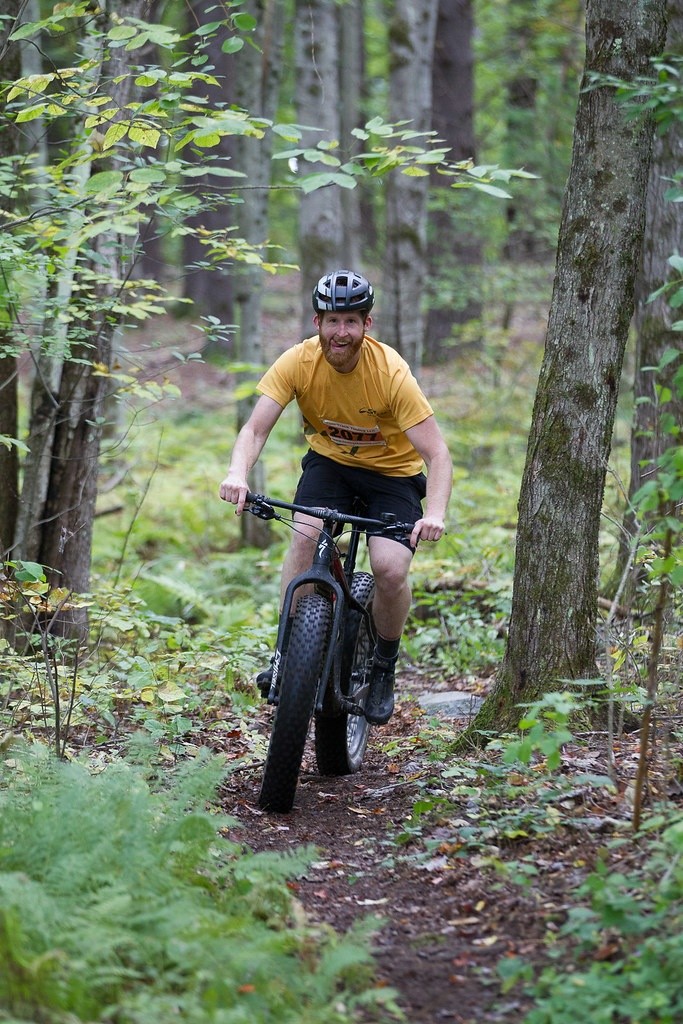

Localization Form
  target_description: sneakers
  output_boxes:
[364,647,400,725]
[257,655,287,695]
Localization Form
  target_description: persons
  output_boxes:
[220,269,453,725]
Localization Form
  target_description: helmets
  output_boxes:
[312,269,375,315]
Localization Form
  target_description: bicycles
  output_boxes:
[238,491,419,815]
